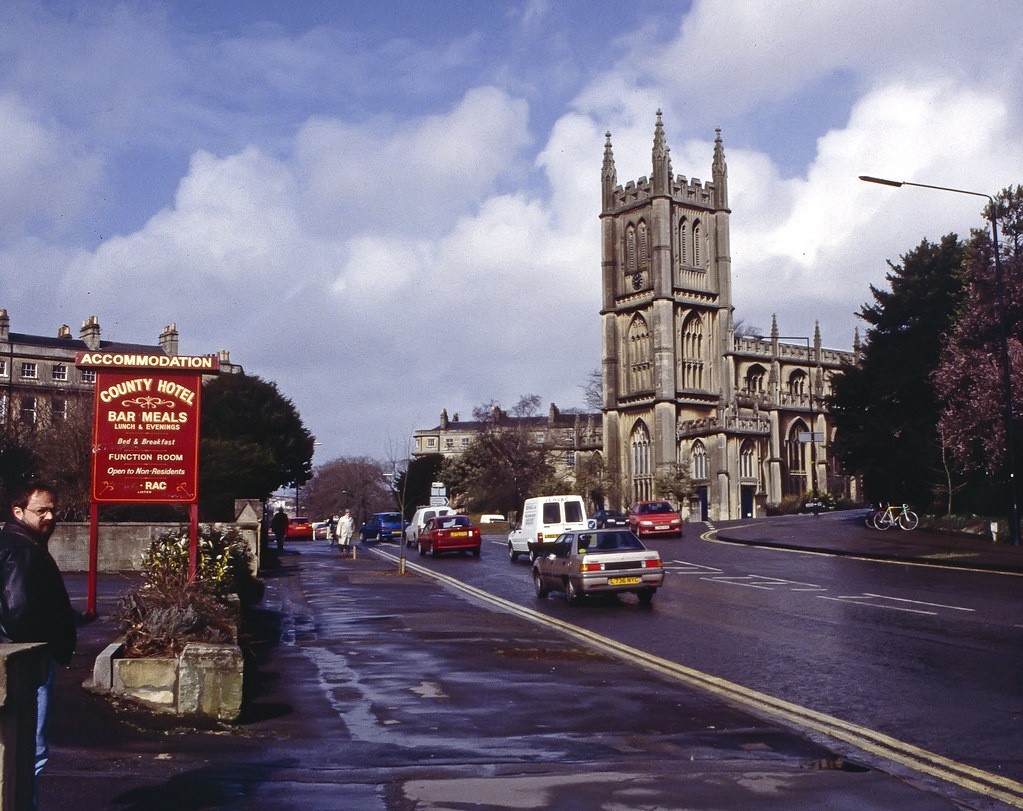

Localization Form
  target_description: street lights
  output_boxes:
[750,330,819,497]
[858,174,1020,539]
[296,442,323,517]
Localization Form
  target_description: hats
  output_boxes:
[278,508,284,511]
[345,508,351,512]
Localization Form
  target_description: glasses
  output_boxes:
[24,505,60,518]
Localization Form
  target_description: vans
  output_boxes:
[405,506,454,549]
[506,495,589,564]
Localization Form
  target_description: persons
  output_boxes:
[272,506,288,552]
[0,482,76,811]
[336,509,355,559]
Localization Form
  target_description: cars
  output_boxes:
[314,523,328,541]
[286,516,313,541]
[629,500,683,537]
[267,527,276,542]
[590,510,631,529]
[531,528,665,605]
[416,515,482,558]
[358,512,410,543]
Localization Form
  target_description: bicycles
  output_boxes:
[864,503,878,528]
[873,498,919,531]
[326,522,339,545]
